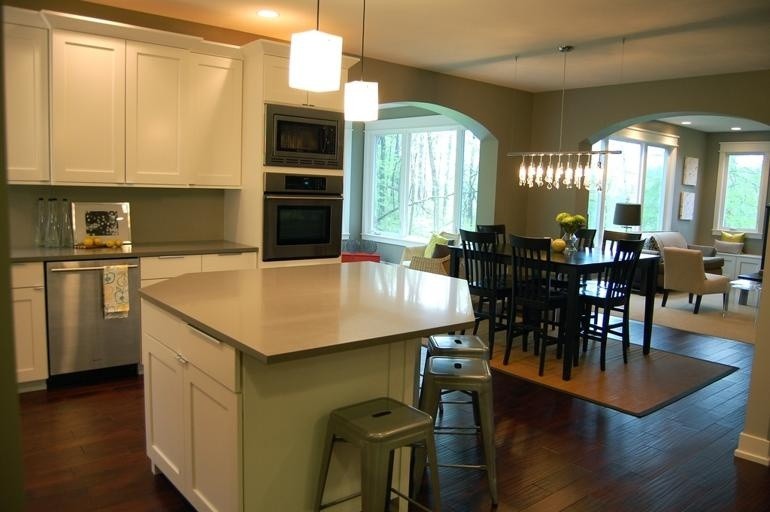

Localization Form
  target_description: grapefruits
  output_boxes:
[551,239,565,252]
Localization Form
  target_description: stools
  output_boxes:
[315,333,498,511]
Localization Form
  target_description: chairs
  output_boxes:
[661,246,731,315]
[399,243,465,279]
[459,225,647,376]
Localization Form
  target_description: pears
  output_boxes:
[556,212,585,225]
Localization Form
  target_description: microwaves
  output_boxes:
[264,103,344,171]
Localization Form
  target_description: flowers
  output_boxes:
[556,212,586,239]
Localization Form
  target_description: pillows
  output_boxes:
[422,232,463,259]
[649,231,688,262]
[714,231,746,254]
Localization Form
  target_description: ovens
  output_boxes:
[262,171,342,263]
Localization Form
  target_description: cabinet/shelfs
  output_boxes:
[3,22,243,186]
[140,298,241,511]
[714,254,762,281]
[138,252,258,366]
[10,262,50,385]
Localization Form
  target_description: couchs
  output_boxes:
[629,231,724,275]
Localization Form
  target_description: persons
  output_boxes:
[86,211,119,236]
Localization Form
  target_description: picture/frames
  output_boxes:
[70,200,133,244]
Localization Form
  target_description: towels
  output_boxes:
[103,265,130,320]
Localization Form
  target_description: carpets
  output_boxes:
[578,280,756,345]
[422,307,740,419]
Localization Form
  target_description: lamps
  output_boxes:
[505,43,622,192]
[287,1,379,123]
[612,203,641,232]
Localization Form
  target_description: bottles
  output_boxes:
[35,197,75,248]
